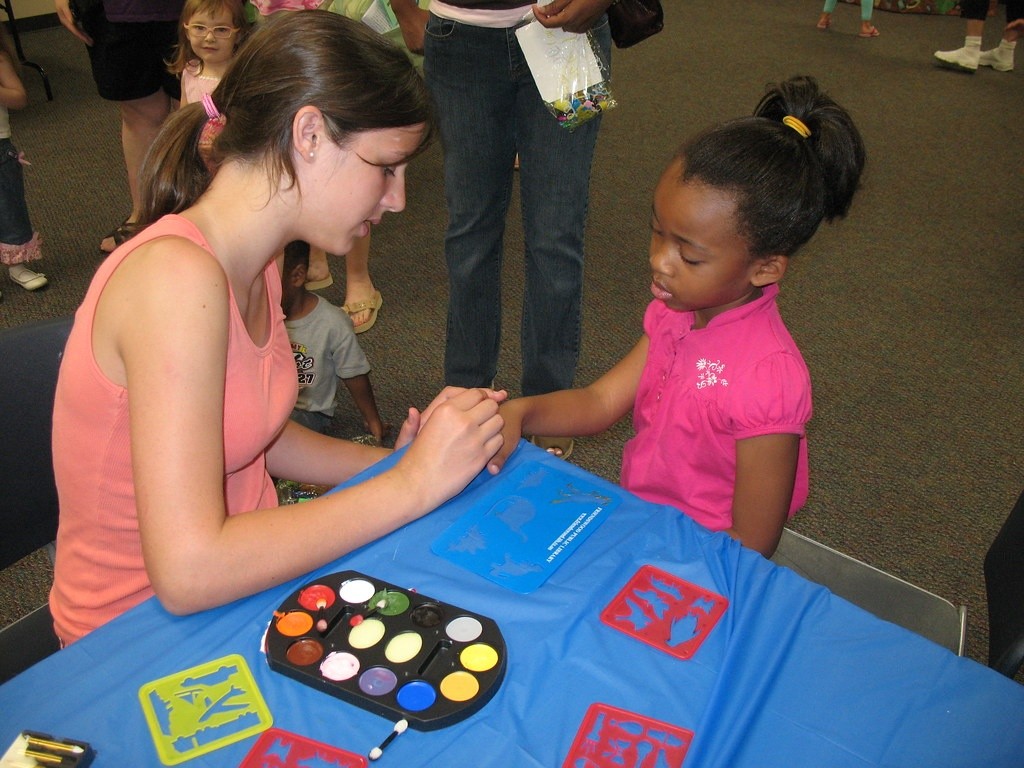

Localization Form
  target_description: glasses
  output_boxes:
[183,24,239,39]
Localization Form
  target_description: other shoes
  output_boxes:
[530,435,574,460]
[9,268,48,290]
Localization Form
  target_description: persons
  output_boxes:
[254,0,431,335]
[281,239,393,445]
[934,0,1024,72]
[0,52,47,292]
[164,0,252,206]
[48,9,509,645]
[53,0,191,253]
[816,0,880,37]
[486,77,868,561]
[389,0,614,461]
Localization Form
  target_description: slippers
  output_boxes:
[859,27,880,37]
[342,290,383,333]
[817,14,832,29]
[304,272,333,291]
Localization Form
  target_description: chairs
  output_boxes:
[0,306,76,689]
[768,521,967,662]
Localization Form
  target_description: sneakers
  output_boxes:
[979,47,1015,71]
[934,47,980,69]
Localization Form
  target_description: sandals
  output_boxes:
[99,214,139,253]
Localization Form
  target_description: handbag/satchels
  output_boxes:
[606,0,664,48]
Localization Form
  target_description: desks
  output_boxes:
[0,432,1024,768]
